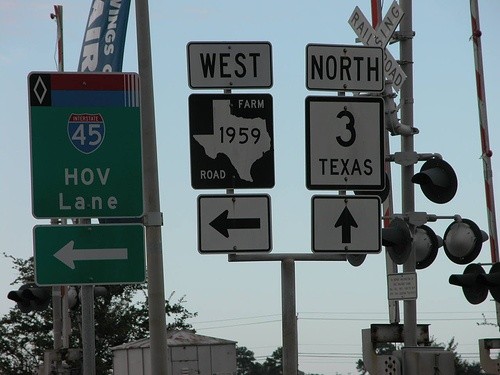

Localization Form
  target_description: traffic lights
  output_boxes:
[411,158,458,205]
[411,225,443,270]
[443,219,488,264]
[448,262,500,305]
[7,282,50,314]
[382,218,411,264]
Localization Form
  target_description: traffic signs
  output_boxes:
[27,72,144,218]
[305,43,385,93]
[305,95,385,191]
[349,0,407,94]
[188,93,274,189]
[187,40,273,89]
